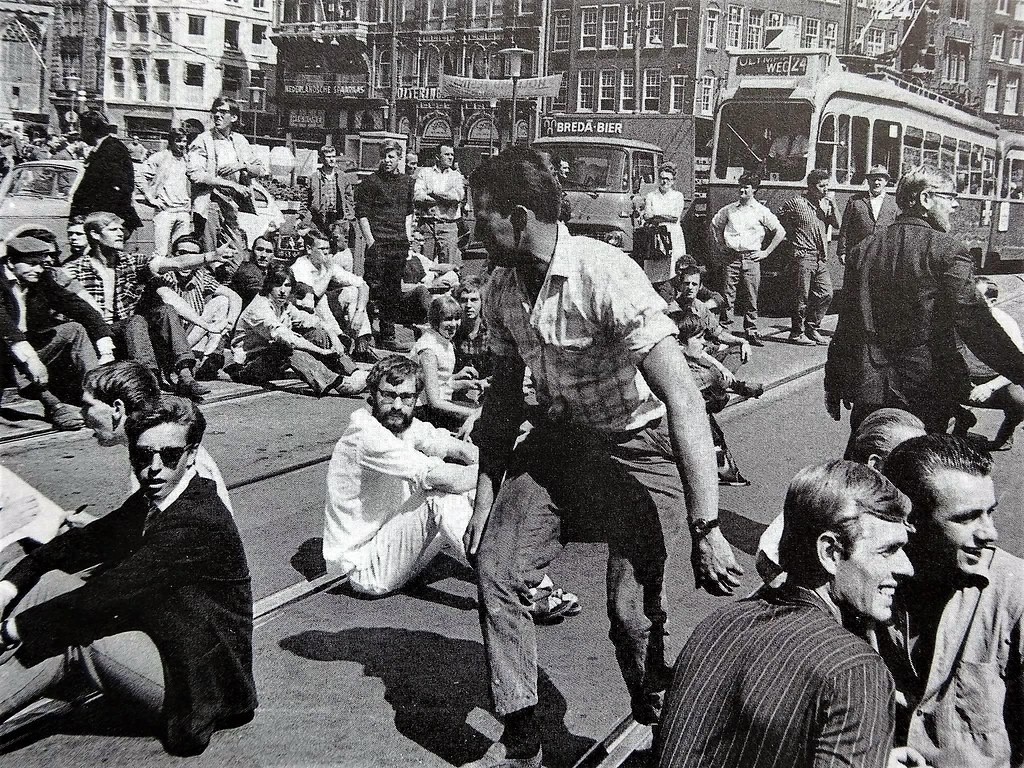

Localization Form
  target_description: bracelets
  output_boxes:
[0,619,16,647]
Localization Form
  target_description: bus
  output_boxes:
[705,49,1024,270]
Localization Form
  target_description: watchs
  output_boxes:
[690,518,721,541]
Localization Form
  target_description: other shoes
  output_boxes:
[194,352,224,382]
[459,742,543,768]
[788,332,817,345]
[335,375,367,395]
[351,341,384,364]
[952,411,977,435]
[745,334,764,347]
[804,330,828,346]
[734,381,762,396]
[174,379,212,396]
[349,369,370,379]
[992,424,1014,451]
[377,337,411,352]
[715,450,748,487]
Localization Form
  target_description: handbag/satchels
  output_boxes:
[833,342,888,406]
[632,220,672,260]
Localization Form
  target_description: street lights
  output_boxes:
[248,86,266,146]
[500,48,535,148]
[63,72,81,125]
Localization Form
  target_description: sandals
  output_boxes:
[529,587,572,621]
[43,403,86,429]
[539,579,582,613]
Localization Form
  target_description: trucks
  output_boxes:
[533,114,713,258]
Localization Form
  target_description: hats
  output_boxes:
[7,236,49,257]
[862,164,891,180]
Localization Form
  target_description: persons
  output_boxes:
[0,96,1023,768]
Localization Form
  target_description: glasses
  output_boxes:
[209,107,230,116]
[926,190,959,201]
[128,442,193,468]
[378,386,418,406]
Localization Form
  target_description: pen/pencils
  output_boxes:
[59,504,88,529]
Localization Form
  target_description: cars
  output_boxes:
[0,160,288,265]
